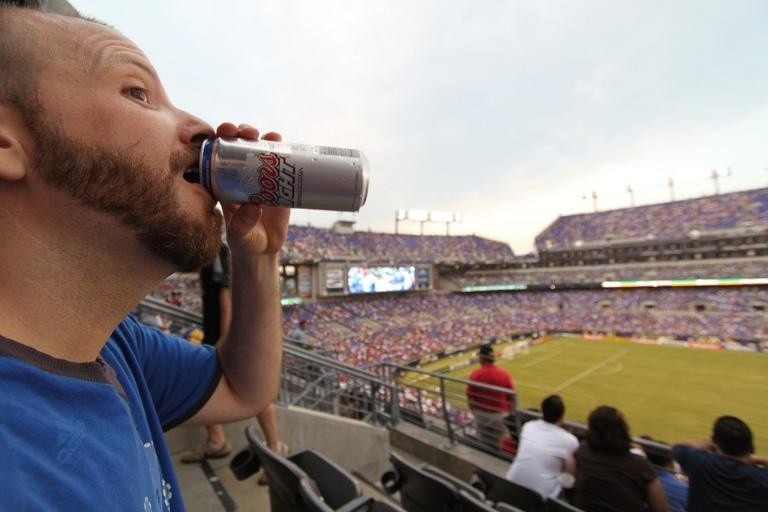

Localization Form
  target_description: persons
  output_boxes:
[565,287,768,351]
[280,368,477,434]
[467,344,515,446]
[500,393,767,511]
[137,274,205,347]
[451,261,767,289]
[0,0,294,512]
[281,292,569,376]
[274,225,513,263]
[180,210,292,486]
[293,320,307,343]
[534,189,766,244]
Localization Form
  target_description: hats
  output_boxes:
[476,347,492,355]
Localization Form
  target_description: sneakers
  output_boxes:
[258,443,290,484]
[181,441,231,462]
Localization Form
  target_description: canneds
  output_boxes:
[199,135,371,211]
